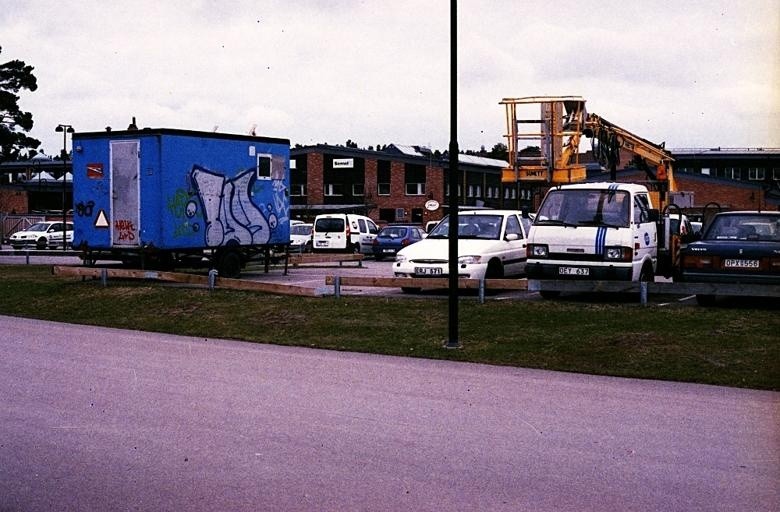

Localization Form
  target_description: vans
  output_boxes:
[9,221,74,250]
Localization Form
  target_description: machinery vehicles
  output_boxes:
[498,95,695,303]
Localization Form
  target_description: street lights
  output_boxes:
[55,124,75,250]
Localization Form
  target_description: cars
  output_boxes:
[679,210,780,307]
[666,213,779,241]
[392,210,549,296]
[31,209,74,215]
[286,214,443,262]
